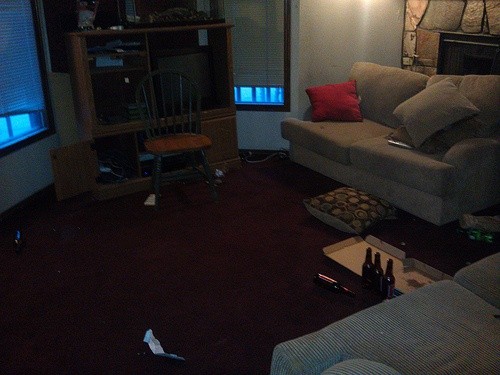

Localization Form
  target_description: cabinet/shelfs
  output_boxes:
[49,22,240,201]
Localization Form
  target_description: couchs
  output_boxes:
[281,61,500,226]
[271,250,500,375]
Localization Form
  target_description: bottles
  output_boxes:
[456,228,493,243]
[312,272,358,297]
[362,247,395,299]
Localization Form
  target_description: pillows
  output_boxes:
[387,77,481,153]
[303,186,394,235]
[304,80,363,122]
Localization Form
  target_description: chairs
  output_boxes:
[135,69,218,210]
